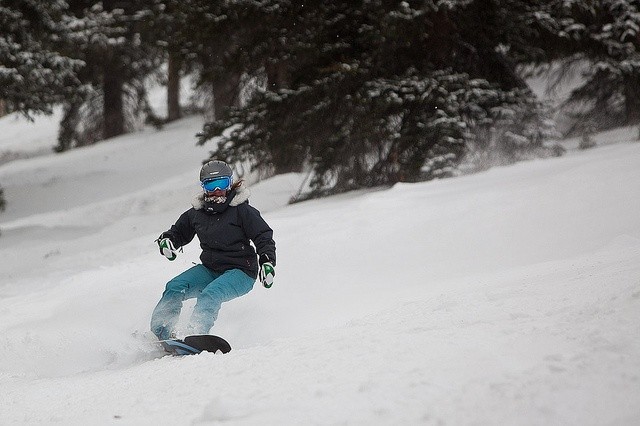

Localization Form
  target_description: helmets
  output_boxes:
[200,160,232,181]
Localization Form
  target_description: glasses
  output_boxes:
[202,178,231,193]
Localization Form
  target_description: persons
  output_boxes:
[149,160,276,342]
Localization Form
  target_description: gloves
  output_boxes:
[259,262,275,288]
[160,238,178,261]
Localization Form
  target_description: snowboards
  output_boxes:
[141,334,231,361]
